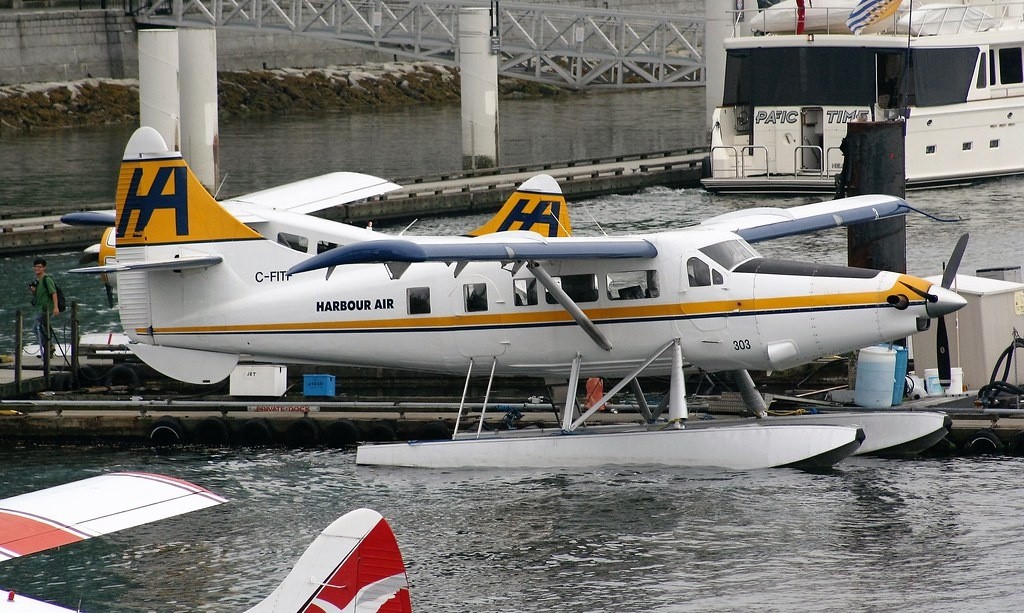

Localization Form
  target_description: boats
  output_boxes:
[356,413,955,471]
[0,364,1024,459]
[698,0,1023,196]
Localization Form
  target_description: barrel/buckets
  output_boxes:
[877,344,908,405]
[855,347,897,407]
[924,368,963,395]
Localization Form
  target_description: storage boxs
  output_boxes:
[303,374,337,396]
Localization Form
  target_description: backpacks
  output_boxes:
[43,275,66,313]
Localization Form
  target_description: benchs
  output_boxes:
[423,303,426,305]
[515,293,523,306]
[618,285,645,299]
[466,297,488,312]
[545,288,613,304]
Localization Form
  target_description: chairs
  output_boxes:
[645,287,659,298]
[410,298,430,314]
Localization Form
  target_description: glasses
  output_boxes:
[33,266,42,268]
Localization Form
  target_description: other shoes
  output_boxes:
[50,348,55,358]
[37,355,41,358]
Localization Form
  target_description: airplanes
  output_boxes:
[59,125,973,385]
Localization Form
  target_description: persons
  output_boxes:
[29,258,60,359]
